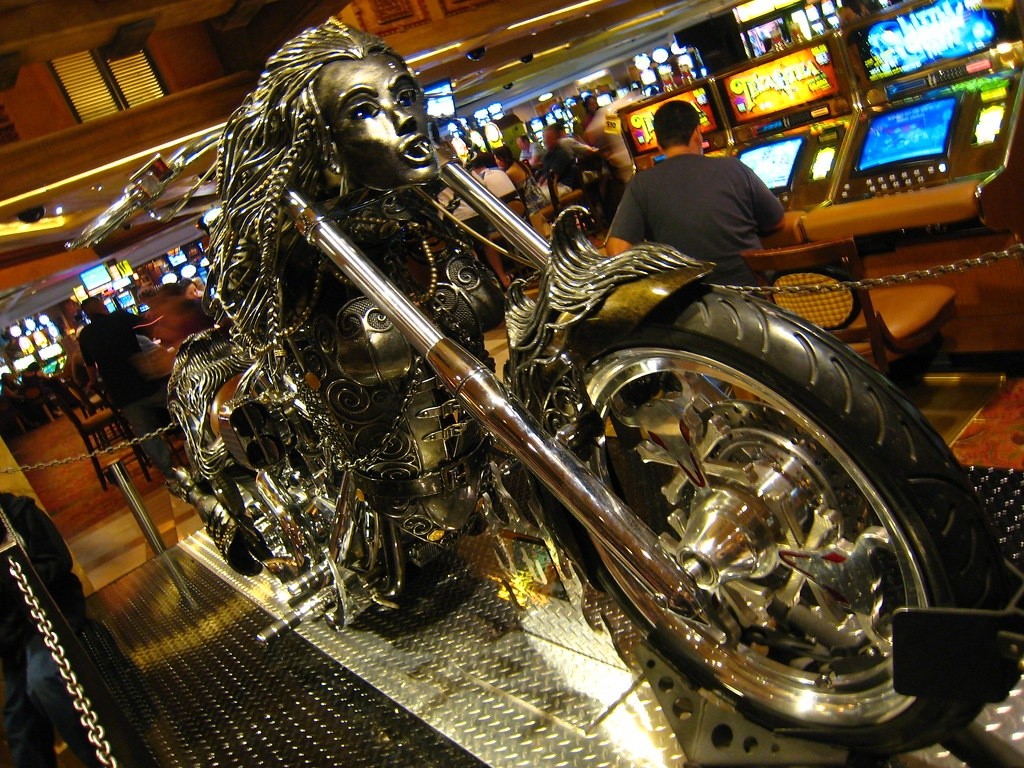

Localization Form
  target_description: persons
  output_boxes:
[420,95,783,397]
[71,279,203,478]
[211,16,449,356]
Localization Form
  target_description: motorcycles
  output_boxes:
[73,22,1012,755]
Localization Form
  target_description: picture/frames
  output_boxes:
[351,0,433,39]
[437,0,500,18]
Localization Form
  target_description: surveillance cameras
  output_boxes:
[199,172,216,183]
[520,54,533,63]
[466,47,486,62]
[123,224,132,230]
[503,83,513,90]
[17,205,46,222]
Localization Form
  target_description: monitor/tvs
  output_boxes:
[165,247,190,271]
[735,136,807,193]
[80,262,115,297]
[422,77,457,120]
[850,89,966,178]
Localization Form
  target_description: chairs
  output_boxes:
[561,155,608,237]
[540,171,576,251]
[743,232,958,374]
[49,364,184,491]
[489,187,535,277]
